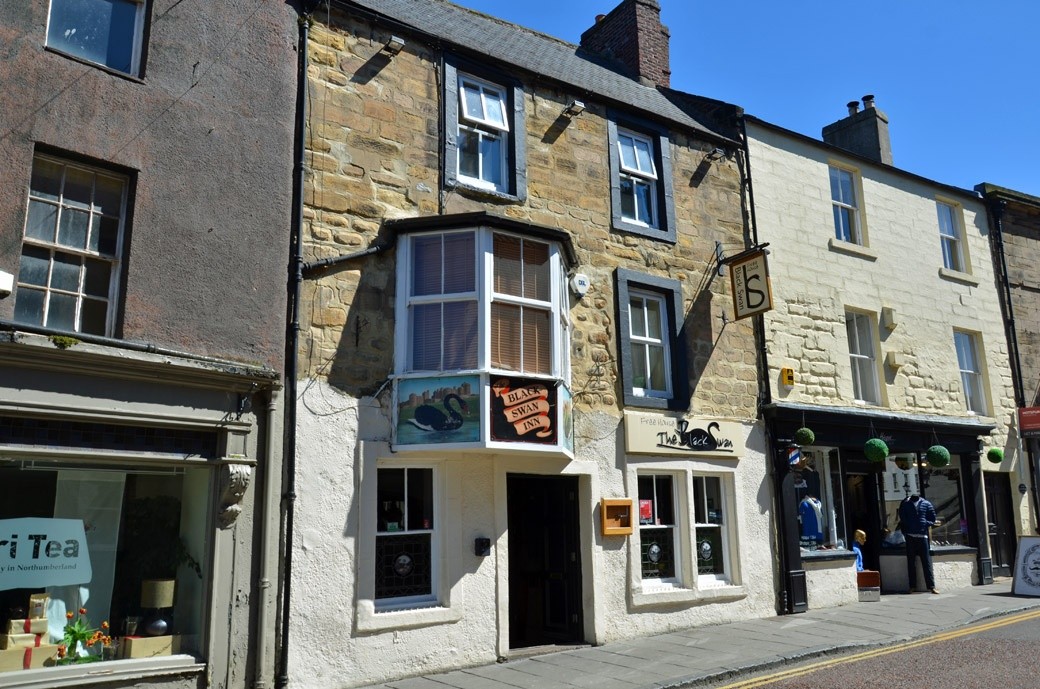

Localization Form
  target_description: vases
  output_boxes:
[68,639,76,656]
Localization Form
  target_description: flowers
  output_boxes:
[57,607,111,659]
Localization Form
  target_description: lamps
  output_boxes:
[140,579,175,634]
[704,148,724,162]
[563,100,585,117]
[384,35,406,56]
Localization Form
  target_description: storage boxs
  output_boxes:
[0,618,58,672]
[118,635,181,659]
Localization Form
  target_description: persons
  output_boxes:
[851,529,870,572]
[898,493,939,595]
[798,489,822,541]
